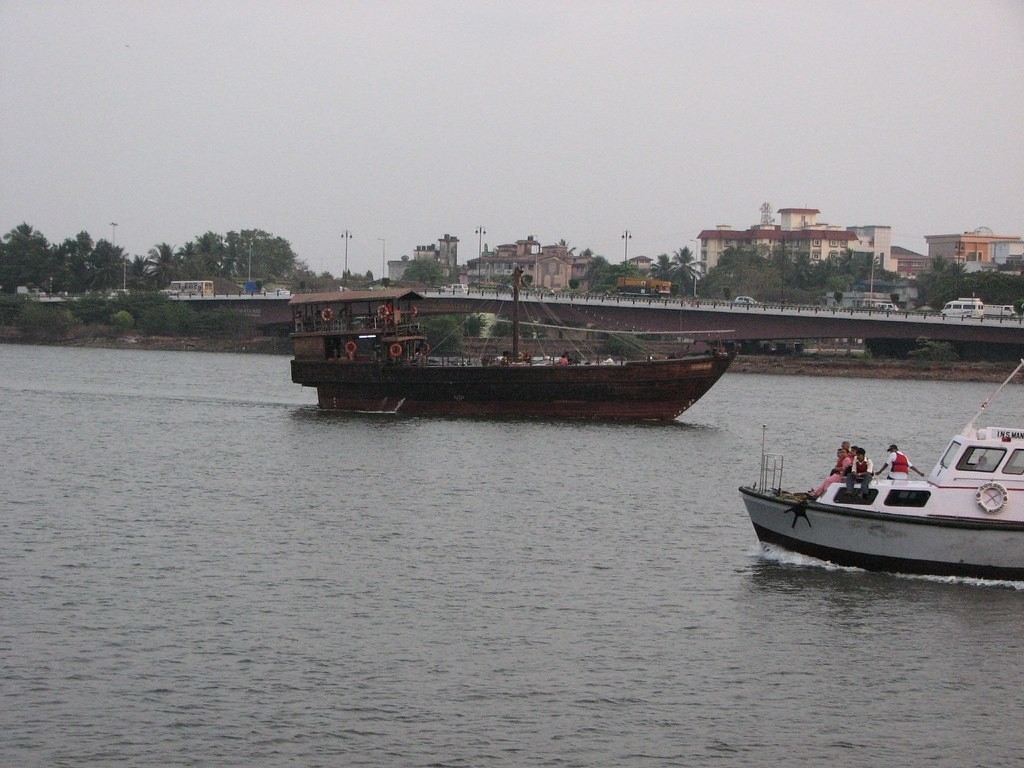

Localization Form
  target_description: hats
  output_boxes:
[887,445,897,451]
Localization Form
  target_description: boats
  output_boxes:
[737,359,1023,583]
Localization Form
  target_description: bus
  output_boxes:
[170,281,215,296]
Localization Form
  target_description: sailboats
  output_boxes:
[288,267,740,422]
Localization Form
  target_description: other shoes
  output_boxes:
[845,488,853,494]
[858,494,863,499]
[804,492,817,500]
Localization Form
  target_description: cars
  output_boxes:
[111,290,127,297]
[496,284,513,290]
[32,288,45,297]
[276,289,291,295]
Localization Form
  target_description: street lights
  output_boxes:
[378,238,385,278]
[622,229,632,298]
[475,225,487,293]
[953,240,965,279]
[870,256,880,309]
[341,230,353,282]
[244,243,251,281]
[690,239,698,296]
[109,222,117,243]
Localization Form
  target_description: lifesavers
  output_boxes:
[975,482,1008,514]
[321,308,333,321]
[410,305,417,318]
[377,305,389,318]
[345,341,356,353]
[390,344,402,356]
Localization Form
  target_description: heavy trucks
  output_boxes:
[616,278,671,299]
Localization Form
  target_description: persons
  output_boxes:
[422,341,430,363]
[875,444,925,480]
[844,448,873,500]
[975,456,994,472]
[500,350,510,368]
[805,441,859,500]
[372,343,381,361]
[414,350,423,368]
[560,354,568,367]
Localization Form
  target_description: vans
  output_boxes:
[440,284,468,292]
[875,303,898,310]
[735,297,757,304]
[939,301,984,318]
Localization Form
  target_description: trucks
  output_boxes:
[242,281,261,294]
[984,305,1018,317]
